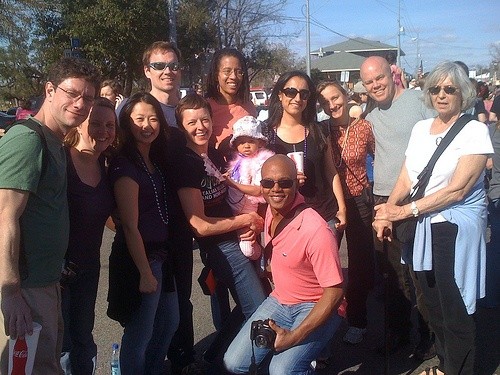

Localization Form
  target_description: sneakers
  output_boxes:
[341,326,368,345]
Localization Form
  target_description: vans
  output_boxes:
[250,90,267,106]
[179,88,197,99]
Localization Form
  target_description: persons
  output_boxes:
[203,48,257,351]
[108,91,180,375]
[16,99,35,120]
[265,70,347,252]
[101,80,125,108]
[223,115,276,278]
[372,62,495,375]
[361,54,438,360]
[114,41,199,373]
[0,55,98,375]
[174,91,267,319]
[223,154,348,375]
[315,65,431,122]
[456,61,500,308]
[0,96,118,375]
[319,80,376,343]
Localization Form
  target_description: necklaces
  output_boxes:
[138,156,169,224]
[272,124,307,174]
[327,115,350,168]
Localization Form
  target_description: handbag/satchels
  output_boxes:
[197,265,217,295]
[389,195,414,242]
[362,184,377,206]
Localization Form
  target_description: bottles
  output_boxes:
[109,343,120,375]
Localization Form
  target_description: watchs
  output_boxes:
[411,201,420,217]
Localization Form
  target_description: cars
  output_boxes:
[0,106,18,130]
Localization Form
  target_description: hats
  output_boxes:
[495,81,500,86]
[411,78,415,81]
[477,79,482,82]
[353,81,367,94]
[229,115,268,145]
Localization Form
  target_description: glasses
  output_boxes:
[281,87,310,100]
[219,68,245,76]
[148,61,182,72]
[51,82,96,106]
[260,180,294,189]
[428,85,456,95]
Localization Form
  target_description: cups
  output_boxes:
[7,322,42,375]
[287,151,304,175]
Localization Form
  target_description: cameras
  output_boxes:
[250,319,281,349]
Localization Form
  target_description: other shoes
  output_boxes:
[315,357,329,370]
[418,365,445,375]
[374,330,409,355]
[181,356,215,375]
[414,339,436,361]
